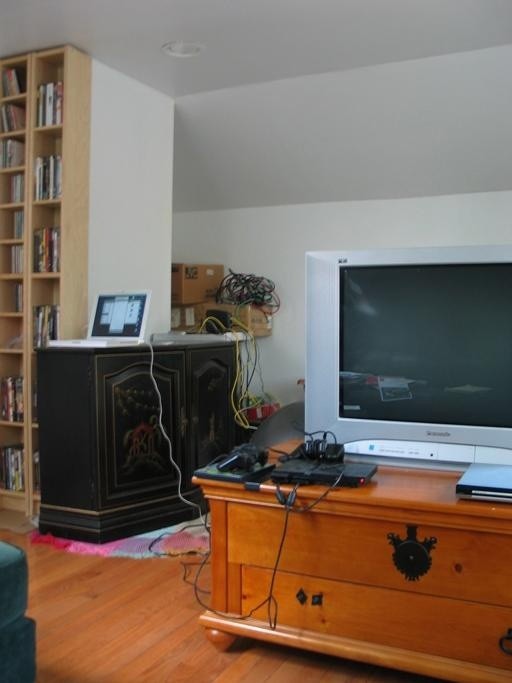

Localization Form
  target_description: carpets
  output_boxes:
[29,510,211,559]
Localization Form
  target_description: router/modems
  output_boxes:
[206,309,232,334]
[271,458,379,487]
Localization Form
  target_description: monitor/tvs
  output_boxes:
[303,242,512,472]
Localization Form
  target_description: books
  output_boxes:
[0,61,64,492]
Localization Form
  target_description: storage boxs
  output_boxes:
[170,263,280,338]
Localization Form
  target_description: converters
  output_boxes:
[325,443,345,462]
[305,440,321,458]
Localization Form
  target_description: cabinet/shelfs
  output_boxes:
[1,49,32,518]
[190,439,512,682]
[27,43,91,518]
[33,342,243,546]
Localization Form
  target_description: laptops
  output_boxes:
[47,290,151,348]
